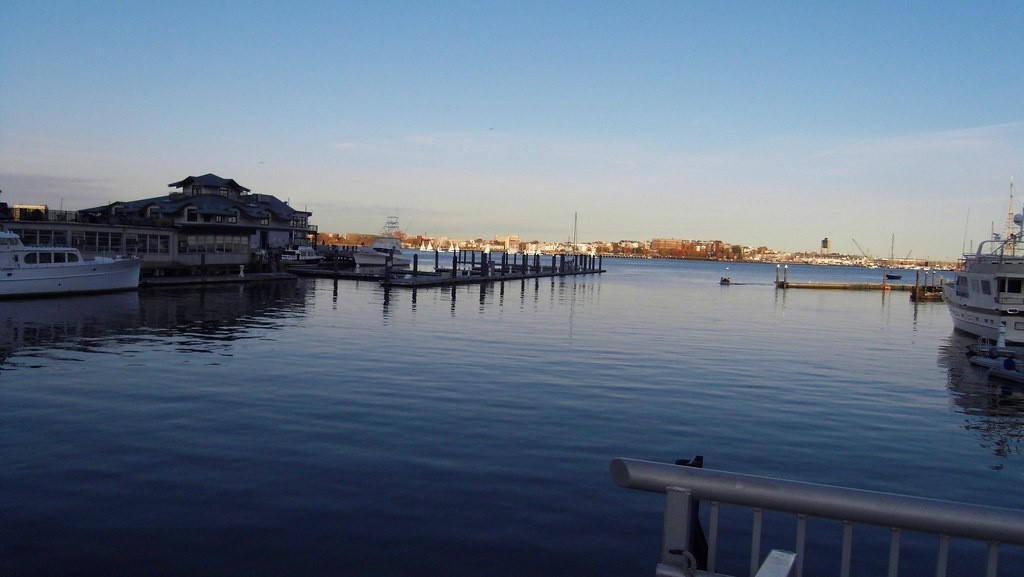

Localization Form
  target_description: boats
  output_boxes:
[940,175,1024,345]
[354,216,413,265]
[718,280,733,286]
[278,244,326,265]
[967,335,1016,357]
[885,274,902,280]
[0,229,145,296]
